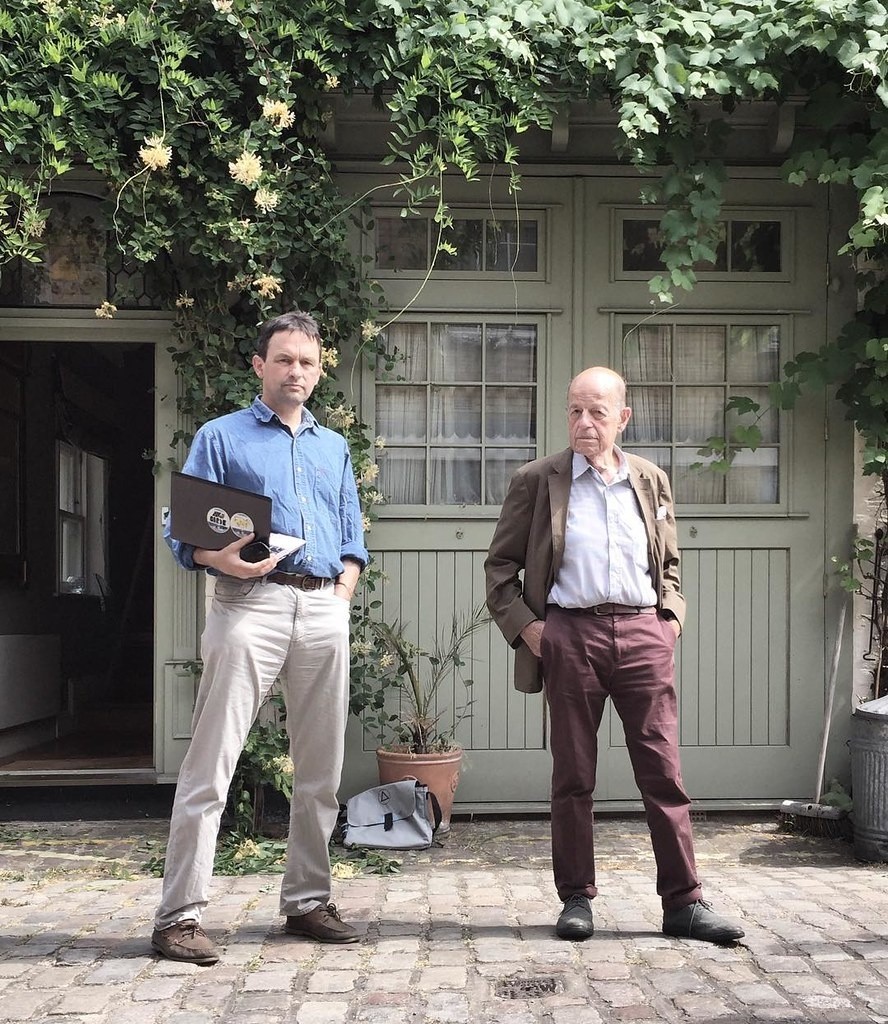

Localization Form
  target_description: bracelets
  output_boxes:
[336,583,352,598]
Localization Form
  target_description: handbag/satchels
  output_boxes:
[343,780,443,850]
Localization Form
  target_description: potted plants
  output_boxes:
[370,601,492,838]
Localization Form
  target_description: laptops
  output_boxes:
[170,470,307,563]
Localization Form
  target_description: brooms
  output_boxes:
[778,600,852,840]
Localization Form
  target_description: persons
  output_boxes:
[483,366,745,941]
[151,311,370,964]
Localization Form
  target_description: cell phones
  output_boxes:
[240,541,270,563]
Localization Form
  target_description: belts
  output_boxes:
[576,603,658,615]
[260,572,336,591]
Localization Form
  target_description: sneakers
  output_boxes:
[151,919,220,963]
[555,894,594,941]
[662,900,746,942]
[286,903,359,944]
